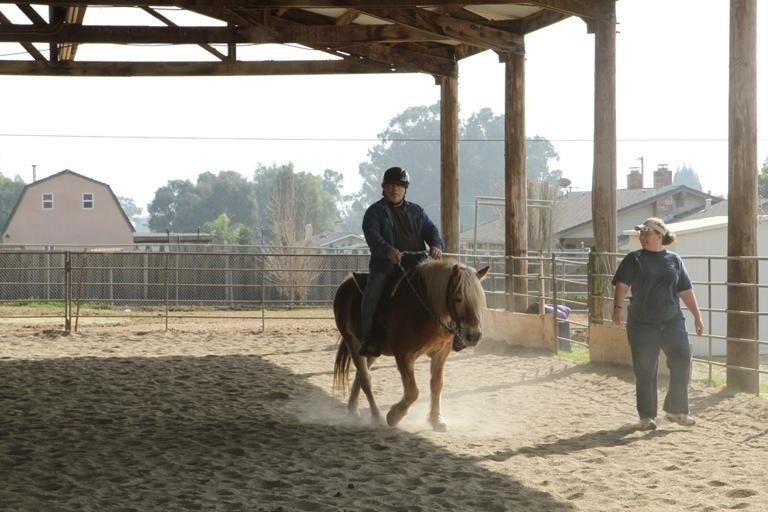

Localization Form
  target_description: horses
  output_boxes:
[332,256,492,432]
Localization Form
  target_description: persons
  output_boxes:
[356,167,468,358]
[612,215,704,429]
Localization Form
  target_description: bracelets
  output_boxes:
[613,304,623,311]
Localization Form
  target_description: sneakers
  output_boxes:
[643,416,658,430]
[665,412,696,426]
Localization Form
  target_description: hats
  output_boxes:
[634,219,666,237]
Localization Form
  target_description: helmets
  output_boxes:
[382,167,410,187]
